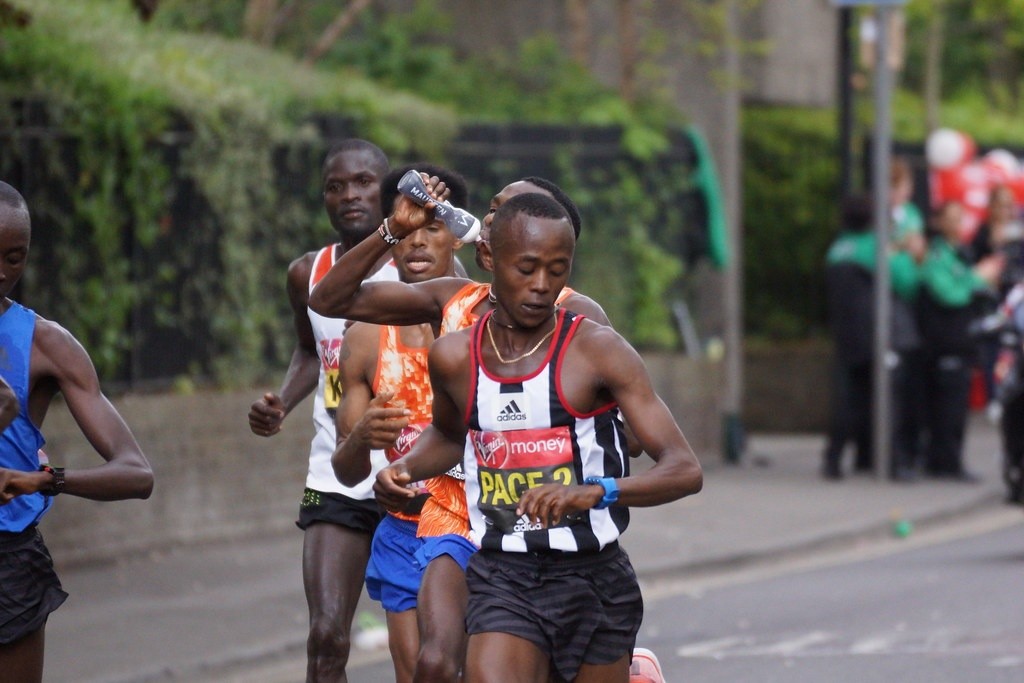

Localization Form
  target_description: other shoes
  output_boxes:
[629,648,666,683]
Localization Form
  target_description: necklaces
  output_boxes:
[487,313,557,363]
[487,287,496,305]
[491,305,558,328]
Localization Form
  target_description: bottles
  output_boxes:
[397,170,482,243]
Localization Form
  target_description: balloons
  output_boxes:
[918,126,1023,243]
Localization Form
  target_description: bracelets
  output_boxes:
[38,464,64,496]
[378,225,400,245]
[383,218,391,236]
[584,474,618,510]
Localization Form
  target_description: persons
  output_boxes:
[307,174,643,683]
[330,163,465,683]
[0,180,154,683]
[372,193,704,683]
[818,159,1024,506]
[248,139,468,683]
